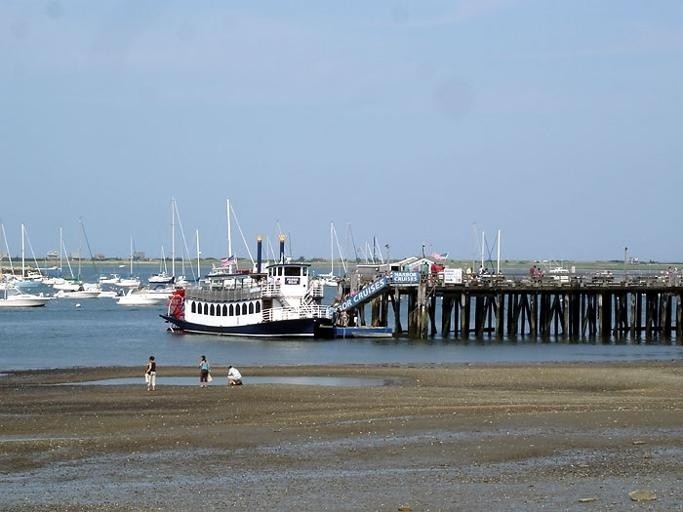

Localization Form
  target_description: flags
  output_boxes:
[431,251,448,261]
[220,254,235,267]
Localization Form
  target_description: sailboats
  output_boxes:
[315,218,574,297]
[0,199,201,309]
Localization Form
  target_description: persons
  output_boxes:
[333,288,358,327]
[198,355,209,387]
[226,366,241,385]
[405,259,544,286]
[143,360,148,384]
[146,356,157,391]
[251,275,326,288]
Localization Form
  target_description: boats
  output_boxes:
[318,312,392,339]
[157,200,337,339]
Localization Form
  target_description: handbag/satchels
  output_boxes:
[206,371,213,382]
